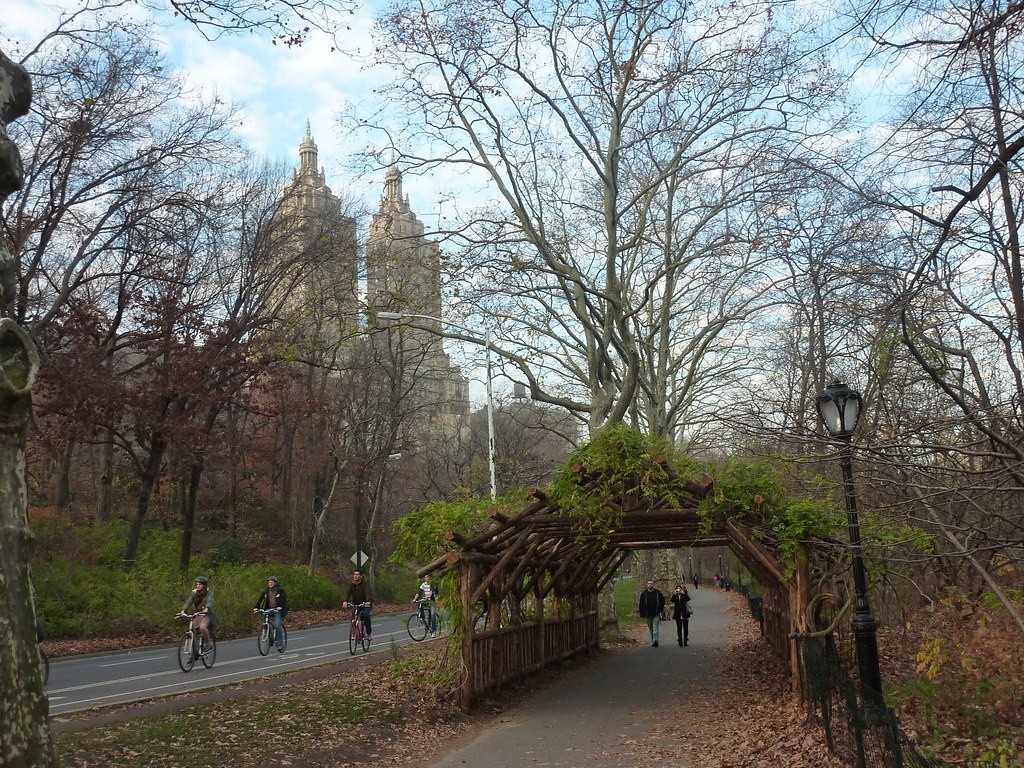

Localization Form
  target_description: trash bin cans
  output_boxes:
[749,596,764,620]
[726,582,730,591]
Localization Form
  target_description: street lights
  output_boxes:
[719,555,722,576]
[817,378,887,730]
[377,311,497,541]
[689,556,692,583]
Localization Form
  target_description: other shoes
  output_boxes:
[684,644,688,646]
[186,660,192,663]
[431,632,436,637]
[654,641,658,647]
[276,646,284,650]
[368,632,373,640]
[205,642,211,651]
[679,643,682,646]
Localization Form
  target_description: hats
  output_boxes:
[267,576,277,582]
[196,577,207,584]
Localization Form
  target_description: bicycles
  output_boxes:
[342,603,372,655]
[174,614,216,673]
[254,608,288,656]
[407,597,441,641]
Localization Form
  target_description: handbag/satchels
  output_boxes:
[685,603,693,618]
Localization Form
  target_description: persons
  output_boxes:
[693,573,699,589]
[714,574,731,591]
[412,574,438,637]
[639,580,665,647]
[180,577,216,663]
[671,585,691,647]
[253,576,287,650]
[343,570,373,641]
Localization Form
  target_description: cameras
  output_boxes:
[676,588,681,592]
